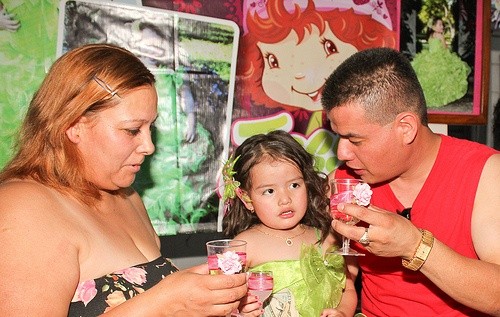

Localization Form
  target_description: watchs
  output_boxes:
[402,228,435,271]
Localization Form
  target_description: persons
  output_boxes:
[220,129,358,317]
[1,44,250,317]
[123,38,213,227]
[410,17,472,107]
[0,0,59,171]
[320,50,500,317]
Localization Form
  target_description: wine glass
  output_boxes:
[206,240,247,317]
[326,179,367,257]
[247,270,274,317]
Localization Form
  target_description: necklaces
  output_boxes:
[255,225,307,246]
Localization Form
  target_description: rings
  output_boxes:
[358,228,370,245]
